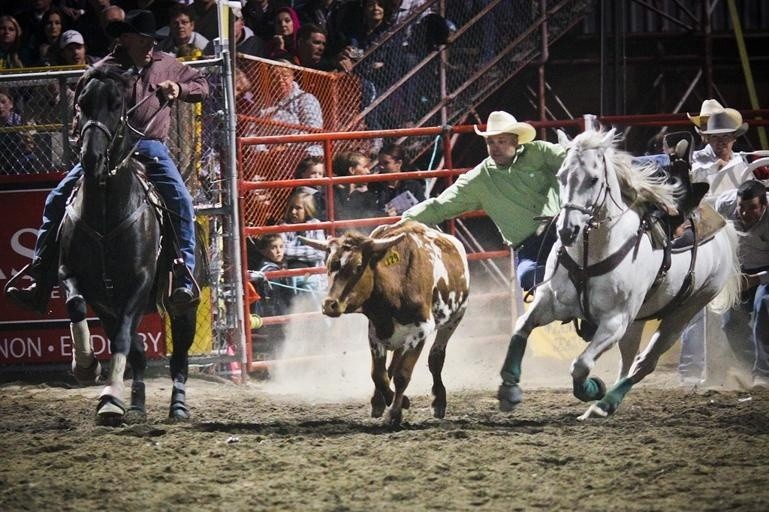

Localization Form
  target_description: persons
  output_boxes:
[3,0,457,313]
[645,96,769,390]
[397,108,565,297]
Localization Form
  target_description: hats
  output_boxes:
[57,28,86,49]
[102,7,167,42]
[472,109,538,147]
[685,97,750,137]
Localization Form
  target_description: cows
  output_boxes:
[296,218,469,425]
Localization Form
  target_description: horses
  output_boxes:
[58,65,205,426]
[494,122,749,420]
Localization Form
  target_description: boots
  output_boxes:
[170,266,200,308]
[6,278,53,316]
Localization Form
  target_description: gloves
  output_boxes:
[156,80,181,102]
